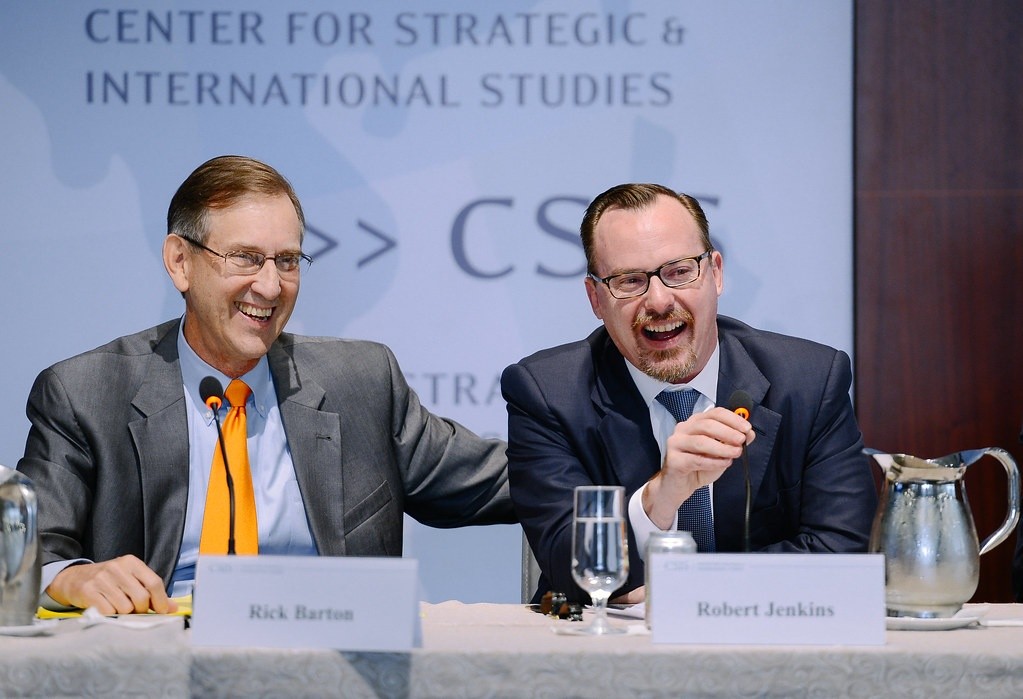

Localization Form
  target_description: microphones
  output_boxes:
[198,376,237,555]
[729,389,754,552]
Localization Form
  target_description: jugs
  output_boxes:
[0,463,45,628]
[861,446,1019,620]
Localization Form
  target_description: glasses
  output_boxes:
[177,233,313,278]
[588,245,713,299]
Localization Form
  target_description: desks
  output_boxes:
[0,598,1023,699]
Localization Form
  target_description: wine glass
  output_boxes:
[570,485,631,635]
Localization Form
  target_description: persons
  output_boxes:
[16,155,518,617]
[499,183,879,606]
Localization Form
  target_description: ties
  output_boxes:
[654,389,716,554]
[198,377,262,557]
